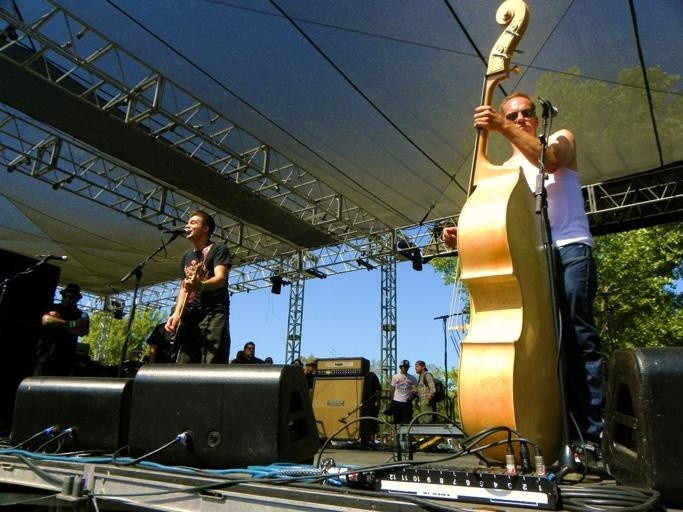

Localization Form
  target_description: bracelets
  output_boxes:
[198,281,206,294]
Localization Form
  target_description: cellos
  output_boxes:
[456,0,563,470]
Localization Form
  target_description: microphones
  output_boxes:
[164,228,191,234]
[540,97,559,119]
[38,254,68,262]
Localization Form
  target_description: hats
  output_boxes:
[399,360,429,372]
[60,283,83,299]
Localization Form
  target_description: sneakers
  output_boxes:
[581,442,599,469]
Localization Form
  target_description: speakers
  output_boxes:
[600,345,683,501]
[310,371,382,445]
[9,374,135,455]
[125,361,320,467]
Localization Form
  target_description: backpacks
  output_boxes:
[424,373,444,402]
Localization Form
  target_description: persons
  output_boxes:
[291,359,316,396]
[232,342,272,364]
[390,360,437,424]
[34,283,89,376]
[146,306,182,364]
[369,372,381,444]
[164,210,232,364]
[442,92,603,443]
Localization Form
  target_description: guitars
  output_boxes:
[167,261,204,345]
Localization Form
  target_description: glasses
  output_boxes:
[506,109,535,121]
